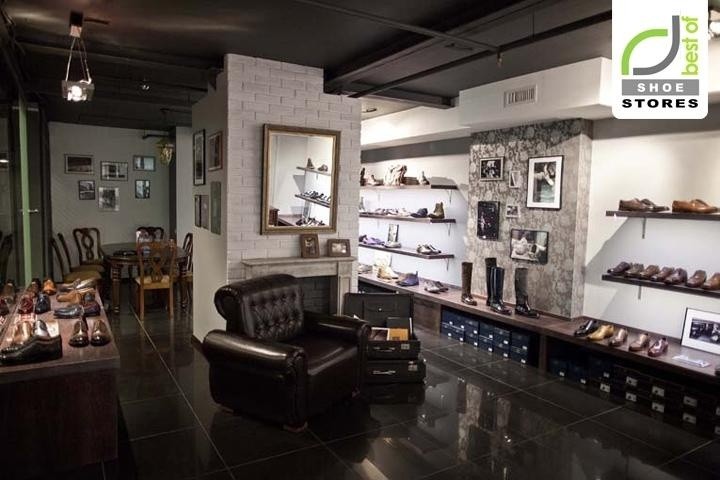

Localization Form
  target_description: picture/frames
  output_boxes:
[299,233,321,259]
[526,155,565,211]
[476,200,501,240]
[504,204,522,219]
[326,238,351,258]
[478,156,506,182]
[189,129,207,187]
[508,170,521,189]
[509,227,550,265]
[63,151,156,212]
[208,131,225,174]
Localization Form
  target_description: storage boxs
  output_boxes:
[542,334,719,441]
[439,310,540,365]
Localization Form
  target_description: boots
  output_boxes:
[461,258,540,320]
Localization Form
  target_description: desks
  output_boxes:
[97,238,188,316]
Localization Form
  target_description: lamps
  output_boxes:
[143,132,175,166]
[59,9,95,103]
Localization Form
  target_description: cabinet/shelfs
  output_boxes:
[1,277,123,479]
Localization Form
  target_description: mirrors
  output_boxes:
[1,99,23,352]
[260,123,342,237]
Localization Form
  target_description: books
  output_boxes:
[370,317,413,340]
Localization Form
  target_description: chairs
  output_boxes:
[47,233,104,289]
[135,225,167,242]
[174,231,193,314]
[71,226,110,268]
[55,232,106,277]
[133,237,179,323]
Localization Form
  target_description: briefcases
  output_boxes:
[343,292,426,384]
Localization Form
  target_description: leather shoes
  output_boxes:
[367,174,379,186]
[358,196,448,293]
[417,171,429,185]
[295,157,331,227]
[606,261,720,290]
[573,318,668,357]
[0,277,111,366]
[621,198,717,213]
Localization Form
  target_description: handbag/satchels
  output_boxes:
[382,163,406,187]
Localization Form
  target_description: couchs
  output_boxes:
[201,273,373,433]
